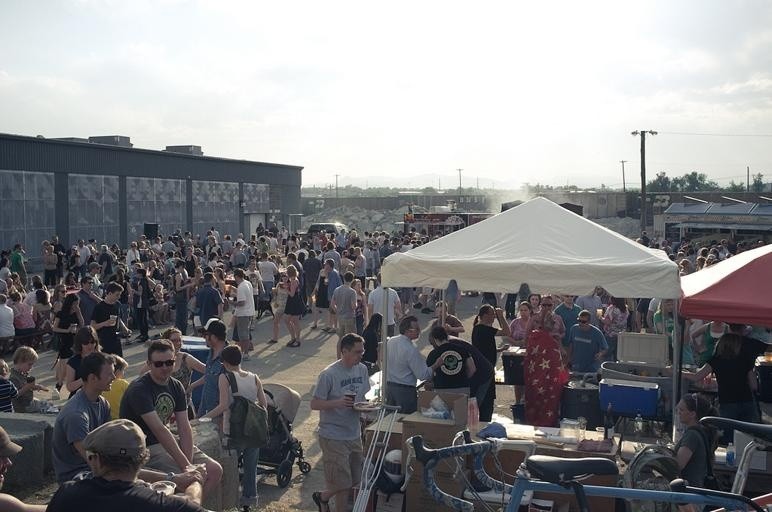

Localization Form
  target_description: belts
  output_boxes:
[387,382,416,388]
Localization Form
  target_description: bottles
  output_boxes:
[605,414,614,440]
[52,388,61,405]
[633,413,643,441]
[725,442,734,468]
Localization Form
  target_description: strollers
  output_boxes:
[236,382,311,488]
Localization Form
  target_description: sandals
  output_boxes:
[312,492,330,512]
[286,339,295,346]
[291,341,301,347]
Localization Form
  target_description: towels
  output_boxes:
[576,439,613,453]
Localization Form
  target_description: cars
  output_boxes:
[307,223,349,236]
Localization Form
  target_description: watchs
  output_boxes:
[166,471,176,481]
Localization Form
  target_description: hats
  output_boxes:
[89,262,102,269]
[83,419,146,456]
[197,318,226,333]
[0,426,23,456]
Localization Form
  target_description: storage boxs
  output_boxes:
[402,470,464,511]
[599,378,662,419]
[400,389,472,476]
[500,352,526,385]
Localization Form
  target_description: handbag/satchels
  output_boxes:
[227,395,270,449]
[703,475,719,491]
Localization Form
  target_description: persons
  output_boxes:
[200,344,268,512]
[426,327,477,395]
[0,427,49,512]
[720,323,771,447]
[54,234,119,352]
[52,352,96,401]
[428,301,465,338]
[429,332,495,408]
[119,339,223,506]
[386,315,444,415]
[385,287,428,314]
[386,226,456,258]
[337,332,384,376]
[0,358,34,414]
[673,392,720,512]
[137,324,209,419]
[187,317,231,443]
[223,222,268,343]
[101,353,130,422]
[0,249,10,358]
[674,318,720,391]
[119,227,222,318]
[10,235,53,345]
[429,278,513,303]
[635,230,767,275]
[269,222,311,347]
[513,283,673,404]
[48,351,117,485]
[311,332,379,511]
[311,228,385,332]
[471,304,512,422]
[10,345,53,414]
[44,418,215,512]
[118,316,137,339]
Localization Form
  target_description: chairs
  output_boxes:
[528,497,555,511]
[463,435,538,511]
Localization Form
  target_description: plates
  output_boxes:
[353,401,381,411]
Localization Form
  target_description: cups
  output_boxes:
[70,321,78,333]
[344,391,356,408]
[595,426,604,439]
[150,480,177,495]
[110,314,117,326]
[597,307,602,316]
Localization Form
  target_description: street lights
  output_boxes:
[332,160,629,199]
[631,130,659,235]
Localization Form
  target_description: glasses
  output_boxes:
[85,450,97,457]
[692,393,697,411]
[576,319,590,324]
[150,360,176,367]
[87,282,94,284]
[80,340,97,345]
[541,304,553,307]
[58,291,67,293]
